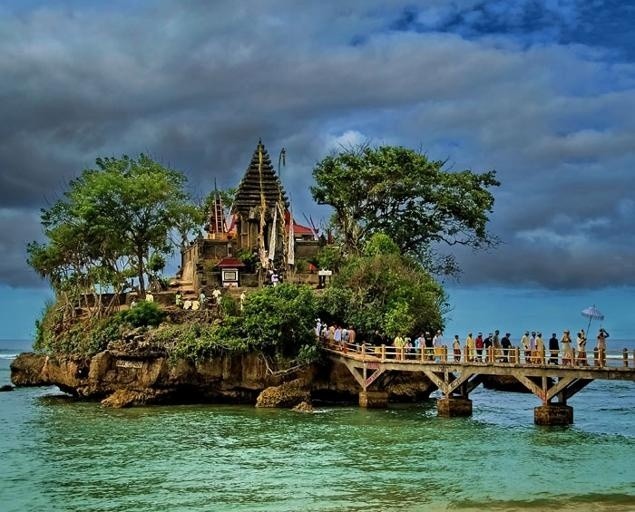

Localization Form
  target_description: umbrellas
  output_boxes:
[577,303,605,344]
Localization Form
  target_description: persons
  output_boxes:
[271,269,281,286]
[174,286,222,311]
[239,290,249,313]
[312,316,611,369]
[145,291,154,303]
[129,297,137,310]
